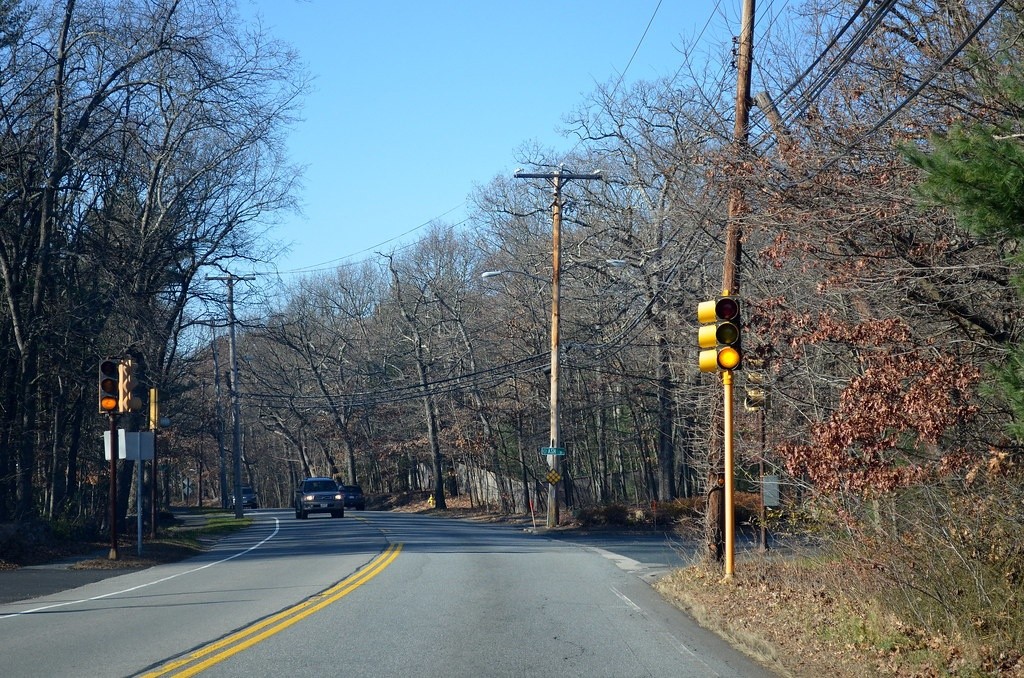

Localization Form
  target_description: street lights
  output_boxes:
[480,256,629,529]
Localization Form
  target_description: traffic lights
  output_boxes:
[697,299,718,374]
[714,292,743,372]
[98,358,119,414]
[149,387,172,431]
[118,358,142,415]
[744,354,772,412]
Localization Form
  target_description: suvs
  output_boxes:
[292,475,346,519]
[226,486,259,509]
[337,483,366,511]
